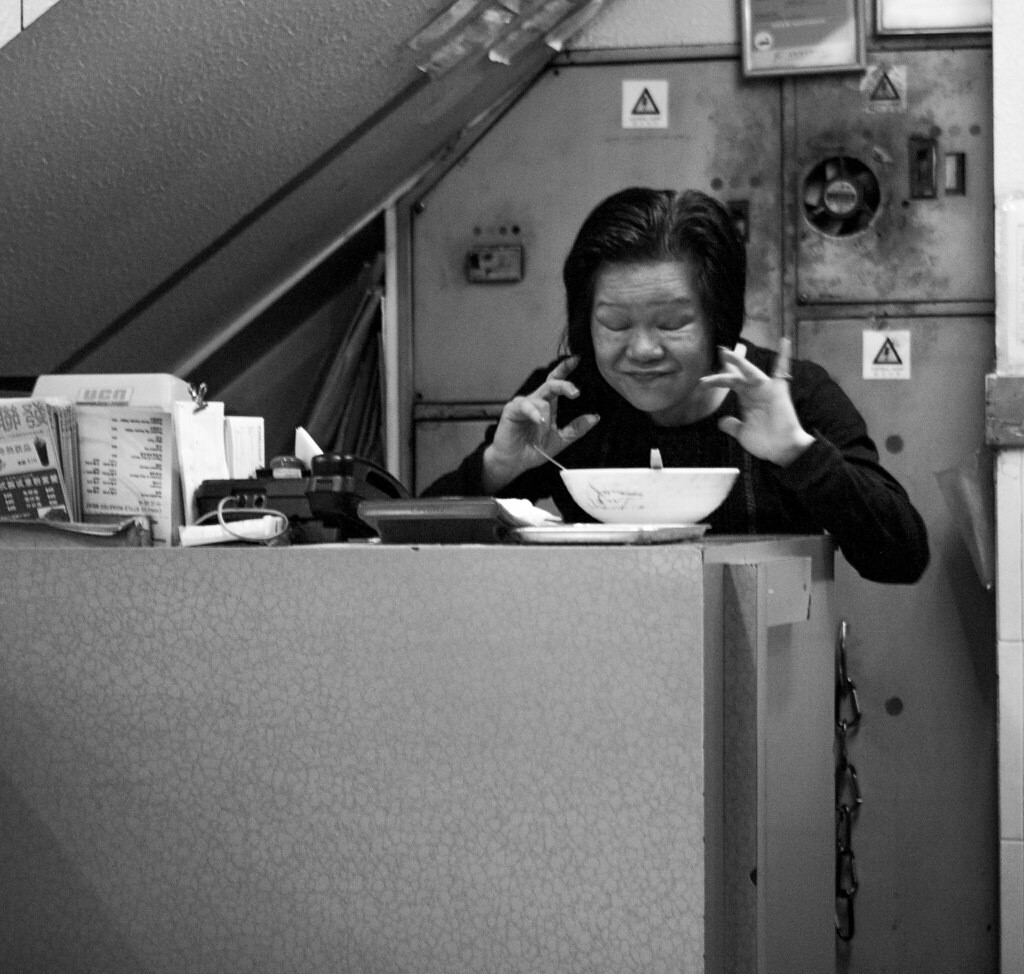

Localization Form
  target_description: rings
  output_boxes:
[769,368,794,381]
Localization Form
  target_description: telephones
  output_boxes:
[194,454,413,538]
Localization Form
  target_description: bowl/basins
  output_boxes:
[560,464,741,524]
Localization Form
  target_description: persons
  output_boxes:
[415,184,935,588]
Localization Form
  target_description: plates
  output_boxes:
[512,525,714,546]
